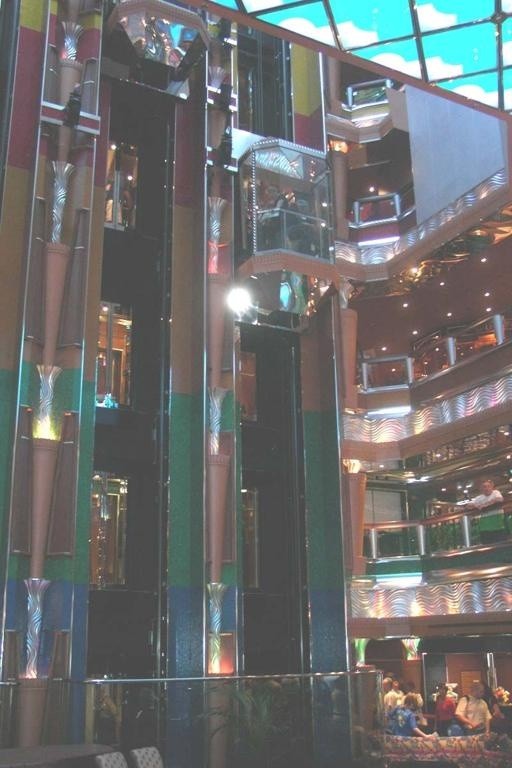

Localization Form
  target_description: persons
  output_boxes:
[382,675,510,740]
[470,480,505,542]
